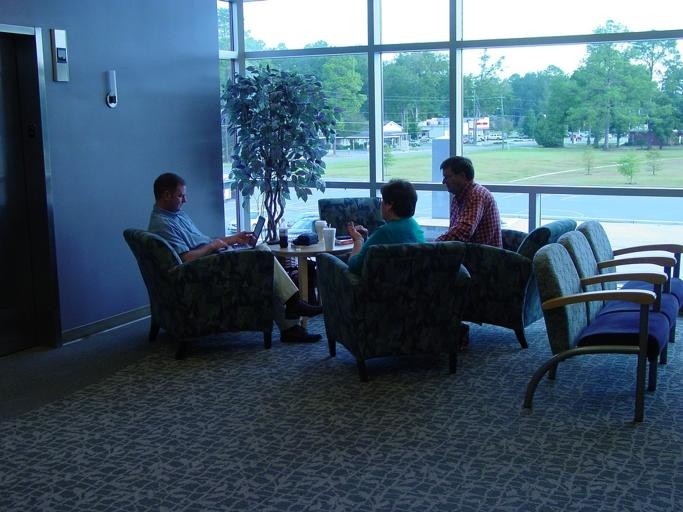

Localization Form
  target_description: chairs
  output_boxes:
[319,198,387,265]
[458,218,578,349]
[525,217,683,423]
[315,240,473,381]
[123,228,277,359]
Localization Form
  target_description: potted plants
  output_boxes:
[215,62,347,271]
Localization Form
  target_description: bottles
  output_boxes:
[279,218,288,248]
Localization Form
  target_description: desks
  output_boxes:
[263,239,355,326]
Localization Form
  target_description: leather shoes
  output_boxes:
[283,297,323,320]
[280,325,323,343]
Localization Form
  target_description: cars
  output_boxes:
[283,214,320,240]
[227,206,289,234]
[406,130,620,148]
[221,174,241,202]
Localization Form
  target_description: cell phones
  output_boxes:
[337,235,352,240]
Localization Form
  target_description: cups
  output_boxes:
[323,228,335,251]
[313,220,326,242]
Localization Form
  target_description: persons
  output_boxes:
[347,177,427,263]
[149,173,323,344]
[430,155,503,249]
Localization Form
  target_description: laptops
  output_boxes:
[218,216,266,253]
